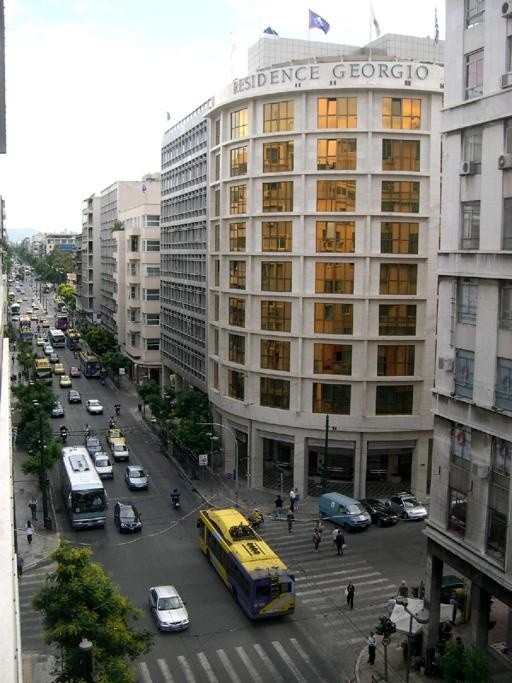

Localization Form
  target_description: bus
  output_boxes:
[59,445,109,529]
[32,356,54,386]
[8,292,34,348]
[50,296,103,379]
[196,506,297,622]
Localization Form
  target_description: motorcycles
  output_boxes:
[173,496,181,508]
[109,420,116,430]
[60,427,68,441]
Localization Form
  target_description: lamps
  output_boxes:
[242,399,258,407]
[296,409,302,416]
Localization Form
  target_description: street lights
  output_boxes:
[395,592,431,680]
[196,419,239,506]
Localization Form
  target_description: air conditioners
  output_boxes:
[460,159,475,176]
[499,73,512,87]
[437,356,453,373]
[468,461,492,480]
[499,0,511,16]
[496,152,511,171]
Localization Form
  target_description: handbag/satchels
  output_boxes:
[345,588,349,597]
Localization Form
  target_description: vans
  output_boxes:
[319,488,372,531]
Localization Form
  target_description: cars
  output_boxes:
[115,502,144,531]
[148,583,190,633]
[8,278,51,348]
[85,398,104,416]
[47,400,65,419]
[42,341,83,389]
[386,491,428,523]
[67,390,82,405]
[359,494,399,527]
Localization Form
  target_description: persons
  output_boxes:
[84,424,89,435]
[346,580,463,674]
[114,401,121,412]
[17,553,24,578]
[274,487,345,555]
[92,495,102,506]
[60,424,68,436]
[109,415,115,426]
[29,495,38,520]
[25,521,33,544]
[170,487,180,504]
